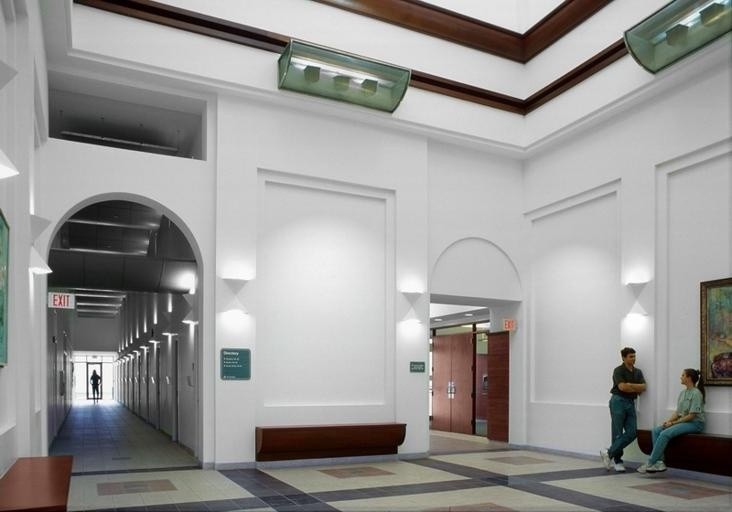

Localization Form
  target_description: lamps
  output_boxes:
[181,310,197,325]
[277,38,411,114]
[28,247,53,275]
[0,148,20,180]
[116,326,178,363]
[623,0,730,75]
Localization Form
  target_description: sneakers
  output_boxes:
[614,463,626,471]
[600,449,610,471]
[637,461,667,474]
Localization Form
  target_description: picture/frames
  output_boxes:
[700,276,731,387]
[0,208,11,367]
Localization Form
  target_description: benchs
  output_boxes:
[1,456,74,511]
[636,428,731,477]
[256,422,406,460]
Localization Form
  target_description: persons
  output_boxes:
[637,368,704,474]
[599,347,647,472]
[89,369,102,398]
